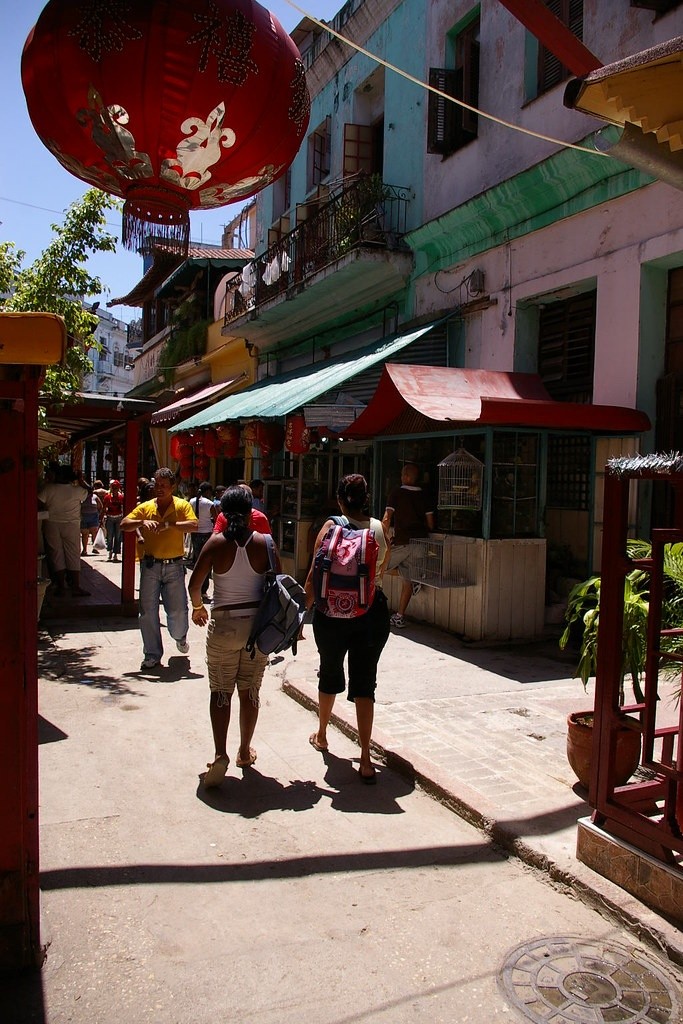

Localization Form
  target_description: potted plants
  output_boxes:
[334,172,391,259]
[558,568,650,792]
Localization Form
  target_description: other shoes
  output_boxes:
[72,587,92,597]
[108,559,113,562]
[81,552,88,556]
[177,638,189,653]
[92,549,99,554]
[141,658,160,669]
[56,587,66,598]
[113,559,122,562]
[202,597,211,604]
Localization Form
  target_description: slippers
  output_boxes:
[359,764,377,785]
[204,755,229,788]
[237,746,256,768]
[309,734,328,751]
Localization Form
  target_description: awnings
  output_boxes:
[165,314,454,431]
[149,373,247,423]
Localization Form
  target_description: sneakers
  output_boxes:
[412,582,423,596]
[390,614,406,629]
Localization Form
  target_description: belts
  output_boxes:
[152,556,183,564]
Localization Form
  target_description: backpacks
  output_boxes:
[313,516,380,619]
[245,534,308,655]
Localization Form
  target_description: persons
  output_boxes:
[302,473,392,782]
[381,463,433,629]
[135,477,278,604]
[37,465,124,595]
[119,466,199,670]
[188,484,283,785]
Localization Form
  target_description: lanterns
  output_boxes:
[171,426,237,482]
[284,415,310,456]
[20,0,312,260]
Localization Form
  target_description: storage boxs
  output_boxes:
[280,479,329,519]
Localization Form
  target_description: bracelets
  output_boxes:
[193,604,203,610]
